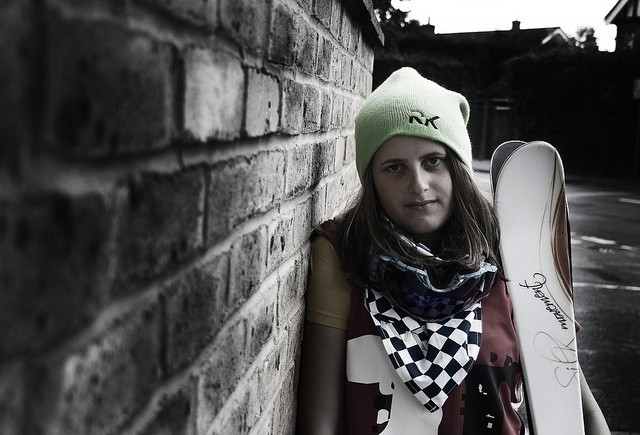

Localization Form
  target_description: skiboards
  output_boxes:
[490,140,585,435]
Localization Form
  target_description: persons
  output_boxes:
[295,65,612,435]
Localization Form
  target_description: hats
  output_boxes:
[354,66,473,187]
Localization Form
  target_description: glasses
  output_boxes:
[376,252,498,323]
